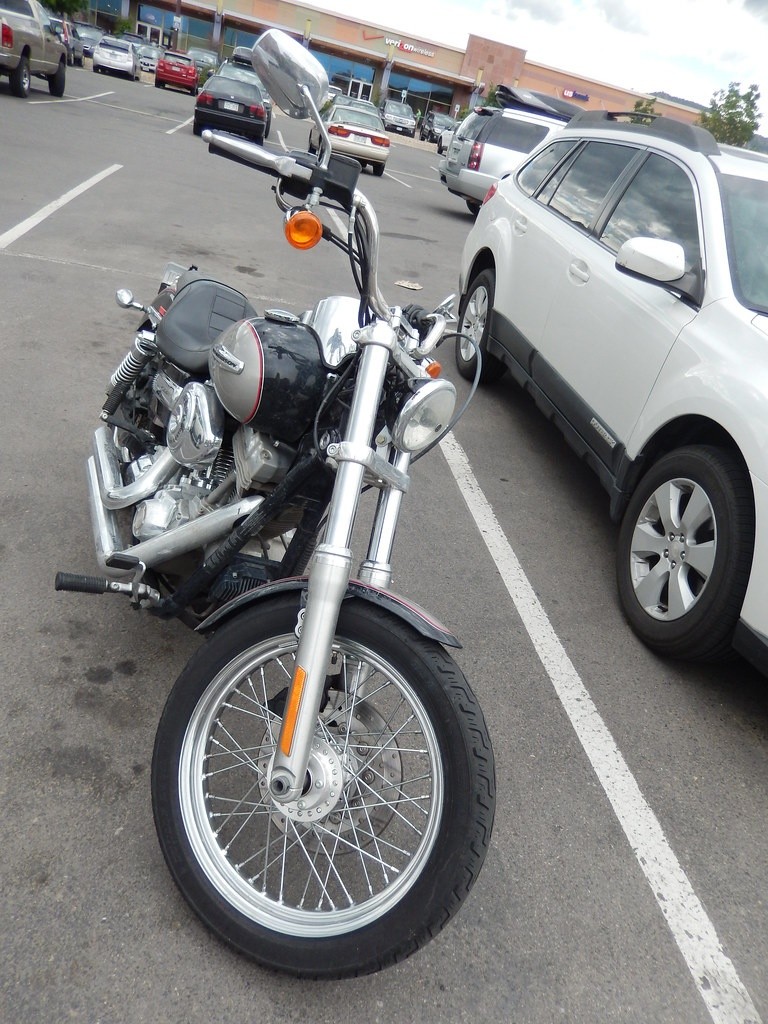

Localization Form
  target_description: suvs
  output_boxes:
[438,83,587,215]
[0,1,70,98]
[448,108,768,688]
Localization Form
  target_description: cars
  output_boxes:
[308,104,392,176]
[326,83,461,156]
[48,12,276,138]
[193,72,268,148]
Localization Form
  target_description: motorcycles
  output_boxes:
[53,25,500,980]
